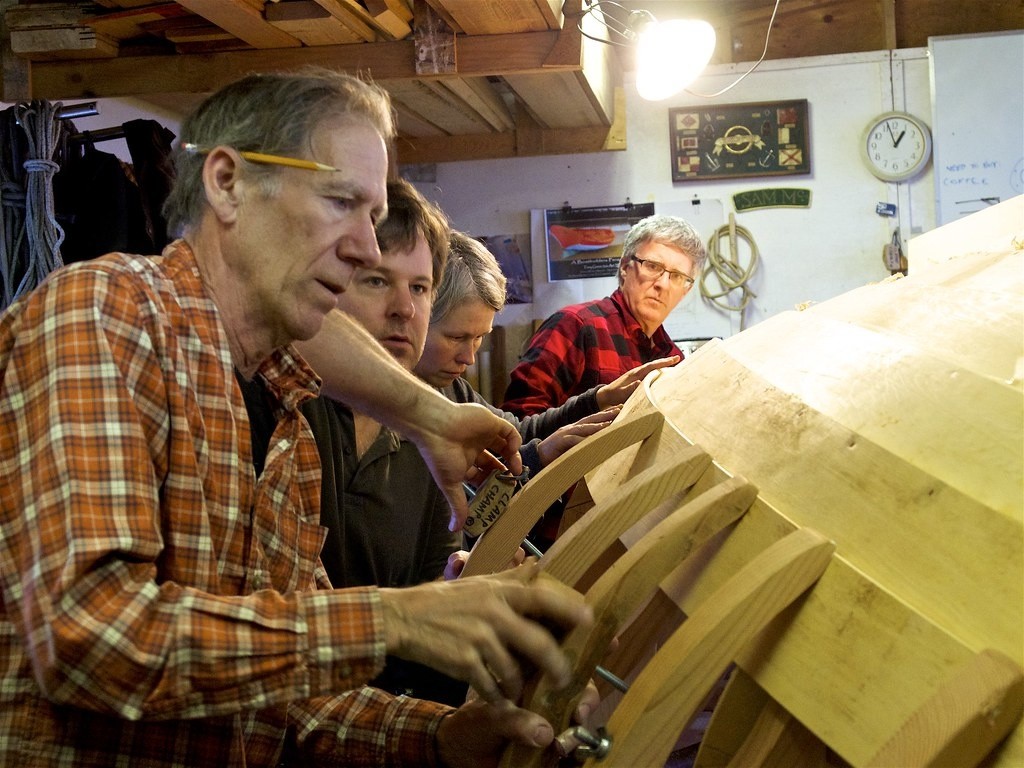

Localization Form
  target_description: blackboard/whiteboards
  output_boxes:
[927,29,1024,228]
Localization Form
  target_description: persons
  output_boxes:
[0,64,619,768]
[498,213,710,555]
[408,228,681,682]
[276,178,524,710]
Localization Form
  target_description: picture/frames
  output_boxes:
[668,98,811,183]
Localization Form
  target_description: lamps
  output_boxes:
[570,0,717,102]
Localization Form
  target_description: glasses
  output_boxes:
[630,254,695,294]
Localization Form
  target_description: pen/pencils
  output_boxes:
[181,142,342,172]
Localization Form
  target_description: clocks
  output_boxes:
[859,111,933,182]
